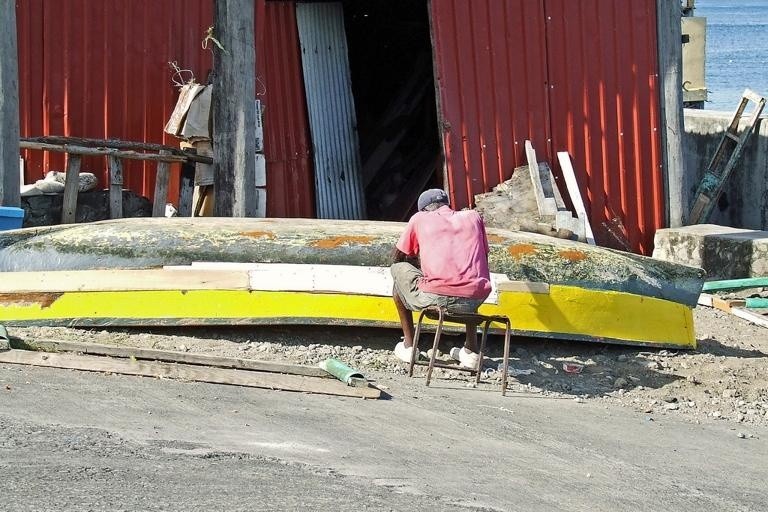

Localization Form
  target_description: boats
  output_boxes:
[0,213,710,355]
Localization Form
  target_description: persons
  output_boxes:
[390,188,491,369]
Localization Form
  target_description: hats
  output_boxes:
[417,189,448,211]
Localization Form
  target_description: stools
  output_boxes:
[408,306,512,396]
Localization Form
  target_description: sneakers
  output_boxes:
[395,336,420,363]
[450,347,479,369]
